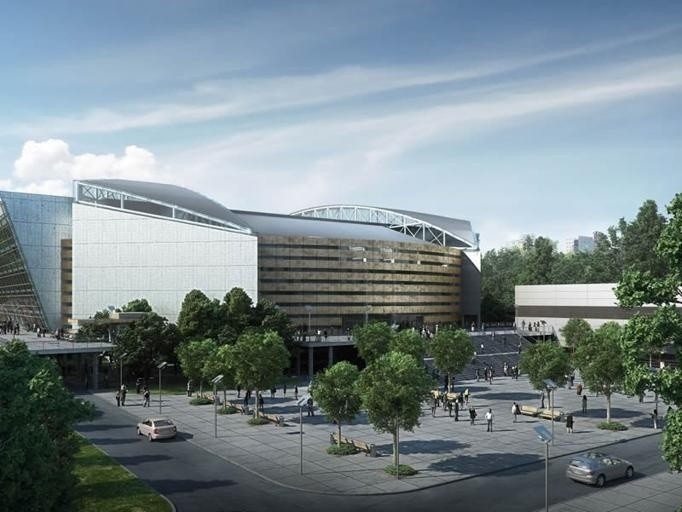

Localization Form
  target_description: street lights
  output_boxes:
[533,425,554,512]
[295,394,313,475]
[542,378,557,440]
[305,305,312,331]
[118,352,128,387]
[541,320,547,343]
[211,374,225,438]
[363,305,372,325]
[157,361,169,414]
[514,303,520,327]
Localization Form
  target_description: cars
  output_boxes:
[565,452,635,488]
[136,417,178,443]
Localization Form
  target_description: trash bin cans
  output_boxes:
[576,384,582,395]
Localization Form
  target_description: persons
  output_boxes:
[121,387,127,405]
[576,382,582,399]
[187,379,194,397]
[566,415,574,433]
[512,320,546,332]
[471,349,521,385]
[259,393,264,410]
[430,387,476,426]
[514,404,520,420]
[121,383,128,390]
[0,315,60,338]
[540,390,546,408]
[143,389,150,406]
[485,408,495,432]
[306,397,314,416]
[581,395,588,413]
[116,388,121,406]
[512,402,518,421]
